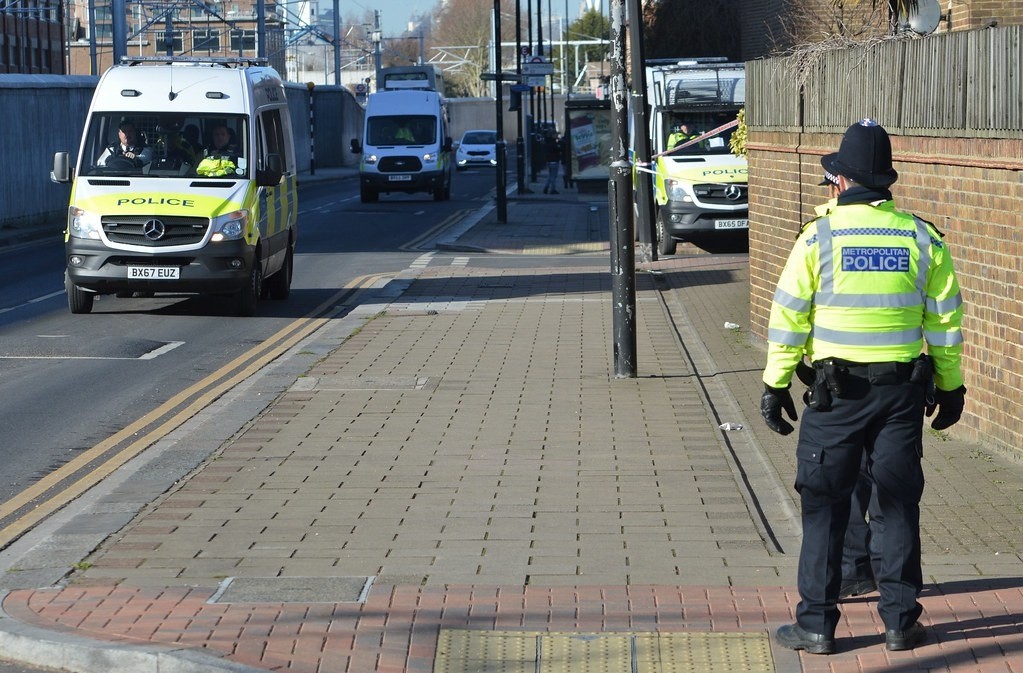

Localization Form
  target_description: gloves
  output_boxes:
[926,385,967,430]
[761,380,798,436]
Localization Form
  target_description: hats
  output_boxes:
[831,120,899,186]
[817,153,841,186]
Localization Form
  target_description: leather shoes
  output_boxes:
[885,621,937,650]
[839,579,877,600]
[775,622,835,654]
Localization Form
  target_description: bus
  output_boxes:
[376,64,451,124]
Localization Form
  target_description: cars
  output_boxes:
[453,129,508,169]
[533,121,558,146]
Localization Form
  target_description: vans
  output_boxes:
[349,89,453,204]
[49,55,299,315]
[627,56,748,256]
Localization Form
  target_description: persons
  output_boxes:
[195,126,238,178]
[394,120,415,143]
[758,119,968,654]
[667,115,711,152]
[543,129,560,194]
[155,124,203,164]
[97,120,154,169]
